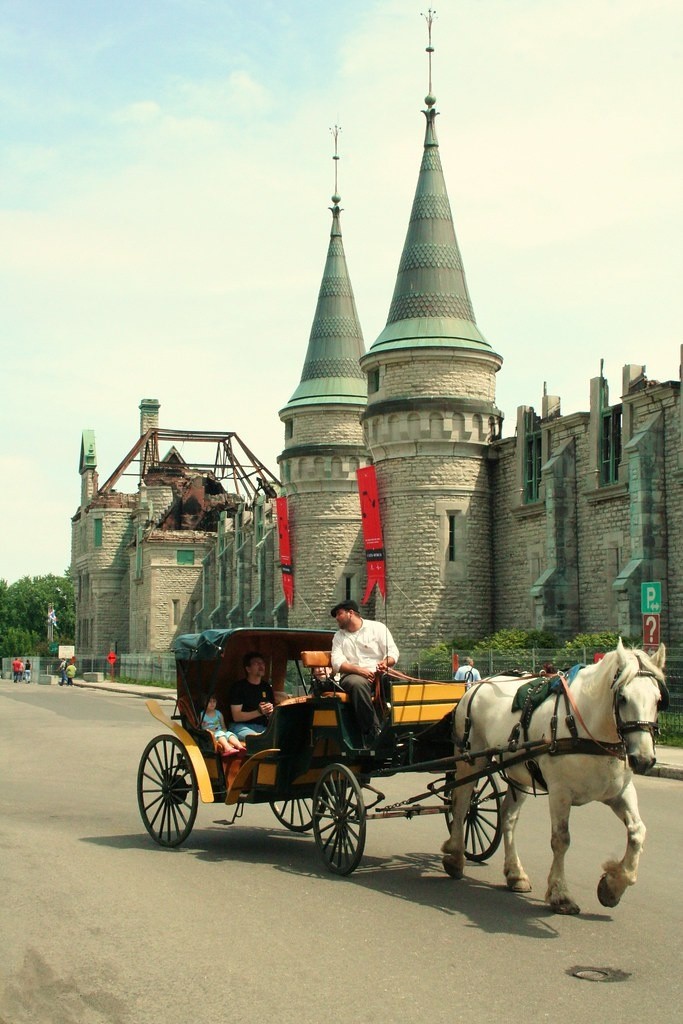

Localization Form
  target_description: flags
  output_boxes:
[47,610,57,627]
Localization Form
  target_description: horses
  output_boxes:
[442,635,667,916]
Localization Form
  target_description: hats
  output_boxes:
[330,599,360,617]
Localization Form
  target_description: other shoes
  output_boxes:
[240,747,247,751]
[222,748,240,757]
[367,727,381,747]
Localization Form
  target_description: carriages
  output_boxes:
[136,624,669,917]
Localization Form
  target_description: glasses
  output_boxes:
[252,661,266,666]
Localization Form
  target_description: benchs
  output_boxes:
[83,672,104,682]
[245,650,379,760]
[179,686,291,789]
[39,675,59,685]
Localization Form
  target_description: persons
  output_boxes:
[453,656,481,691]
[306,666,346,693]
[543,663,556,674]
[330,599,400,749]
[227,652,274,742]
[197,692,247,756]
[12,657,32,684]
[55,658,76,687]
